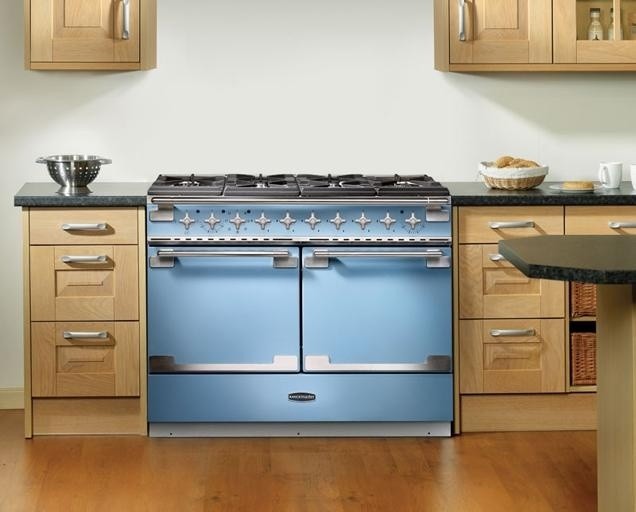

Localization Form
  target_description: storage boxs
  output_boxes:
[571,278,597,316]
[570,330,596,386]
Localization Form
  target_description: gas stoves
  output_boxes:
[147,173,450,242]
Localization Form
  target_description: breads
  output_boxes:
[563,183,593,189]
[494,156,538,168]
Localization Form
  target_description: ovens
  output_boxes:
[147,242,454,422]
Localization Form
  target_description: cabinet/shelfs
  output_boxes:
[19,206,148,440]
[565,206,636,235]
[433,0,636,72]
[458,205,566,397]
[23,0,157,71]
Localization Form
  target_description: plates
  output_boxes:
[549,184,602,194]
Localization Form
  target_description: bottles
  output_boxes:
[587,8,603,40]
[607,8,623,40]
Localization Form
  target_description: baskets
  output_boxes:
[479,161,549,191]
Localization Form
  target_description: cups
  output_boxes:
[598,161,623,189]
